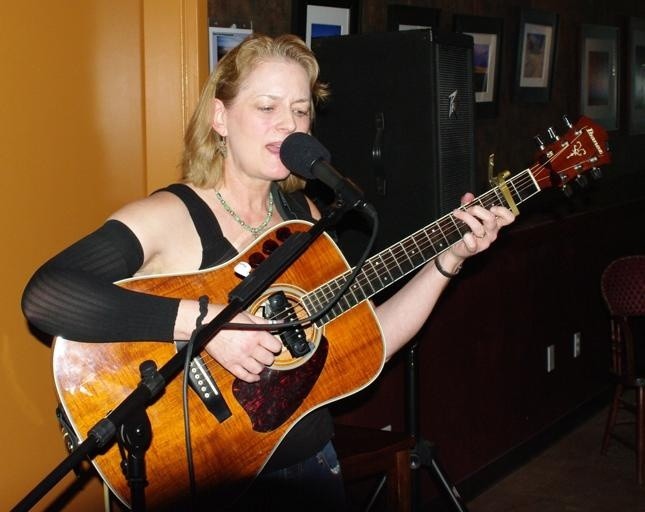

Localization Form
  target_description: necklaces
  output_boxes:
[209,184,274,240]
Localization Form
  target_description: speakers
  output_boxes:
[317,30,476,232]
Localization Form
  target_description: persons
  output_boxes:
[21,31,519,512]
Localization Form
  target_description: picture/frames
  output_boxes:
[292,0,644,139]
[209,26,253,81]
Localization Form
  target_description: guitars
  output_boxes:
[51,116,614,512]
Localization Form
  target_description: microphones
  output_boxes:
[280,133,363,205]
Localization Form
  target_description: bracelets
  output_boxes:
[434,257,460,279]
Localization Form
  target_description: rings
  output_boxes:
[472,231,486,239]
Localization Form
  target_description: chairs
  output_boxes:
[600,255,644,489]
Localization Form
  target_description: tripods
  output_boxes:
[360,327,467,512]
